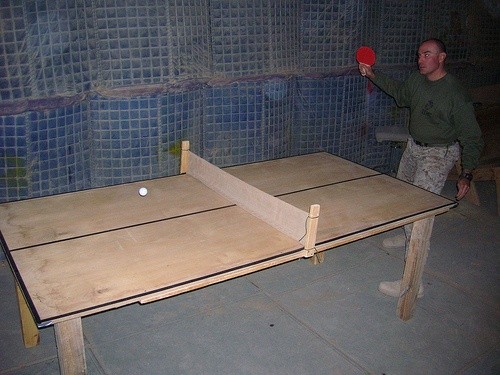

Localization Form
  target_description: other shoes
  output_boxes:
[382,232,406,247]
[378,278,425,298]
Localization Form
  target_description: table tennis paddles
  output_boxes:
[356,46,376,76]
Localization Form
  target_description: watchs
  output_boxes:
[462,172,473,181]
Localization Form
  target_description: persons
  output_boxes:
[359,39,484,297]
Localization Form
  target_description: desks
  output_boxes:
[0,140,459,375]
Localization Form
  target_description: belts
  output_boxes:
[413,139,455,147]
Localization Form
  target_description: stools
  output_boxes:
[372,126,410,177]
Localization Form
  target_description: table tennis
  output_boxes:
[139,187,148,196]
